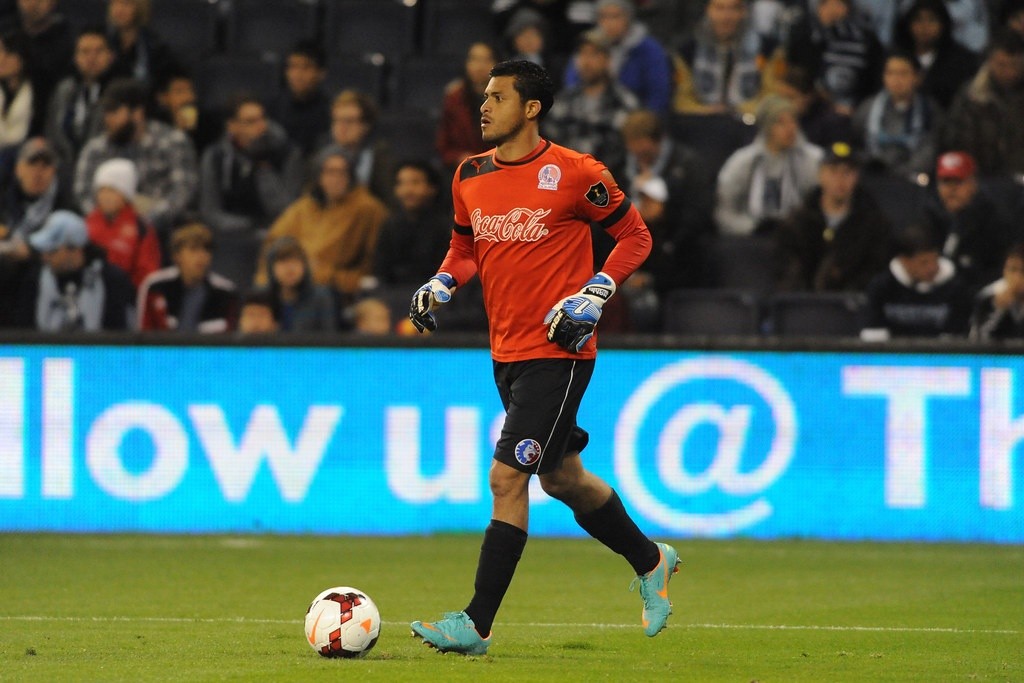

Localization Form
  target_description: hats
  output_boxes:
[27,212,87,252]
[91,159,138,201]
[936,152,978,178]
[823,139,866,171]
[636,179,669,200]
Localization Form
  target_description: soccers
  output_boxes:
[303,586,381,658]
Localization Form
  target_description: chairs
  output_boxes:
[0,0,1024,334]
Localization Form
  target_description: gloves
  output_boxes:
[409,273,457,334]
[542,273,617,353]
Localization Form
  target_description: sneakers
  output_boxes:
[409,610,493,656]
[629,542,683,637]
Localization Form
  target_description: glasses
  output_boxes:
[12,152,58,169]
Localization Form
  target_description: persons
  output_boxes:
[0,0,1024,340]
[408,60,682,656]
